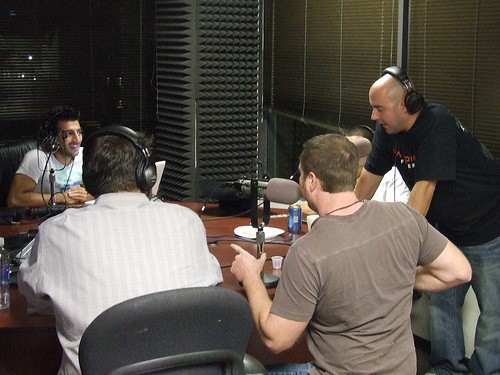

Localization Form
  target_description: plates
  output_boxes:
[233,226,285,239]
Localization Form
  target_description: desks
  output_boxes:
[0,201,315,375]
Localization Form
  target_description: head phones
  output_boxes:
[38,107,78,152]
[83,125,157,191]
[382,65,424,114]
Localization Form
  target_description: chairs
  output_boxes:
[0,140,39,208]
[78,286,268,375]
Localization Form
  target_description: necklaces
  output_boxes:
[325,202,360,216]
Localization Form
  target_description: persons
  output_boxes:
[354,72,500,375]
[346,126,421,302]
[230,134,473,375]
[5,103,95,208]
[16,126,224,375]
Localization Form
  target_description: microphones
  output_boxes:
[233,178,301,205]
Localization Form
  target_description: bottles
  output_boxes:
[0,237,10,310]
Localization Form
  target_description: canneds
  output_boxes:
[287,204,302,234]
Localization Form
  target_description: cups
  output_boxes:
[307,214,319,232]
[271,256,283,269]
